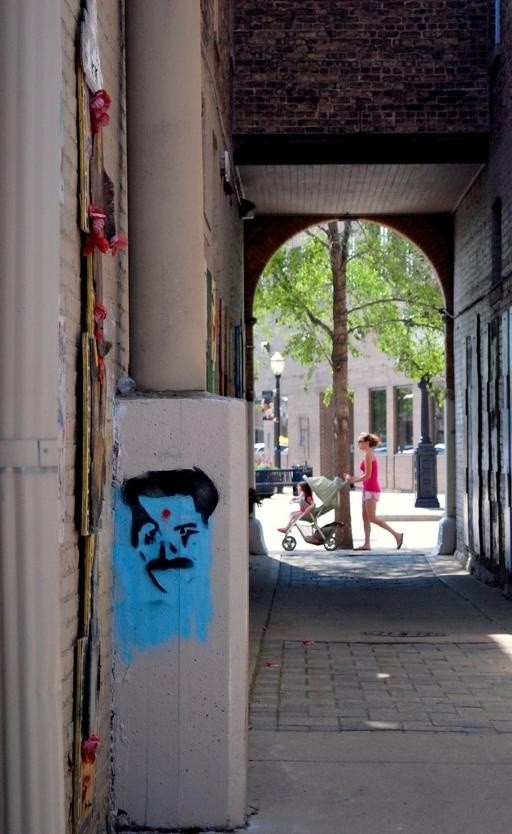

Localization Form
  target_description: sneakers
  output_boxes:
[396,532,403,550]
[353,546,371,551]
[277,528,292,534]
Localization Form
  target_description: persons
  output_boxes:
[275,480,318,534]
[341,431,404,552]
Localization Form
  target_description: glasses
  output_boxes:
[358,440,364,443]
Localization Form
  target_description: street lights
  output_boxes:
[268,351,287,494]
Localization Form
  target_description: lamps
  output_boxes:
[240,196,256,221]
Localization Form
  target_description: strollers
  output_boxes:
[276,472,358,553]
